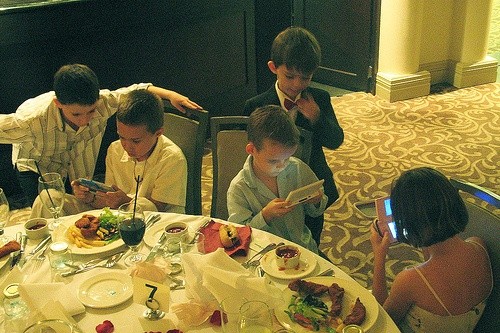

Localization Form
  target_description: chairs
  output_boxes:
[211,115,315,222]
[158,101,209,216]
[450,176,500,333]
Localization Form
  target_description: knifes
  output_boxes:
[19,235,27,259]
[189,220,209,244]
[144,231,166,263]
[20,234,52,268]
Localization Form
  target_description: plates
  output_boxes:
[53,210,130,255]
[259,249,318,279]
[142,220,194,250]
[0,243,10,270]
[77,272,134,308]
[275,275,380,333]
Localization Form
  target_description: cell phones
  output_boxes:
[78,178,115,195]
[376,196,408,244]
[283,178,324,207]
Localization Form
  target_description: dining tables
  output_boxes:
[0,210,403,333]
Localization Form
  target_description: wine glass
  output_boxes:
[0,190,10,243]
[118,203,147,267]
[38,172,65,230]
[162,237,183,274]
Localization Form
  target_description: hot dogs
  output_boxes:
[218,223,240,249]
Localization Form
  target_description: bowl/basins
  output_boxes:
[164,221,189,242]
[275,245,301,267]
[25,218,48,239]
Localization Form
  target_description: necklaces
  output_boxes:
[134,137,160,183]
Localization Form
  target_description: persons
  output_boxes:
[1,63,204,208]
[227,105,333,265]
[368,167,495,333]
[242,25,347,250]
[28,87,190,230]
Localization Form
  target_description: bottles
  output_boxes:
[48,241,73,284]
[1,284,30,319]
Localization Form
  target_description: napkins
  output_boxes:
[180,249,286,320]
[17,282,85,319]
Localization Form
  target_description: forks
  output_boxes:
[63,252,124,269]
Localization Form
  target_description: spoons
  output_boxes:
[250,242,284,266]
[59,253,123,278]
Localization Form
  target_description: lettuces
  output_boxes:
[97,206,123,244]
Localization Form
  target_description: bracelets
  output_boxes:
[86,194,97,206]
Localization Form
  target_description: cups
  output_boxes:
[218,296,249,333]
[22,318,73,333]
[237,300,274,333]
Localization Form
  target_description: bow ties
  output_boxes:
[284,98,299,111]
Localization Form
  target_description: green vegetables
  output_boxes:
[284,294,336,333]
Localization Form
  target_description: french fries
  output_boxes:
[67,225,106,249]
